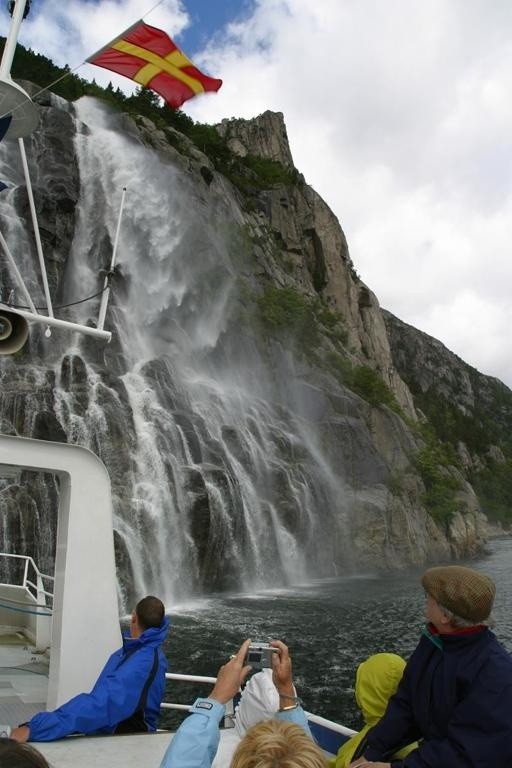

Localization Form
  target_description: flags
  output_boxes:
[87,19,222,114]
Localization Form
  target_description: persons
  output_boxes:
[157,636,325,768]
[328,652,419,768]
[348,565,511,767]
[10,595,170,744]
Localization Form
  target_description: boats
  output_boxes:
[0,0,364,766]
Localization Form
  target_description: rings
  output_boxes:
[229,654,236,659]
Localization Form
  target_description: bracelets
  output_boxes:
[280,702,299,711]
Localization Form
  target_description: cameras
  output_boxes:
[246,642,281,668]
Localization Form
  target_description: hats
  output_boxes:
[421,566,496,622]
